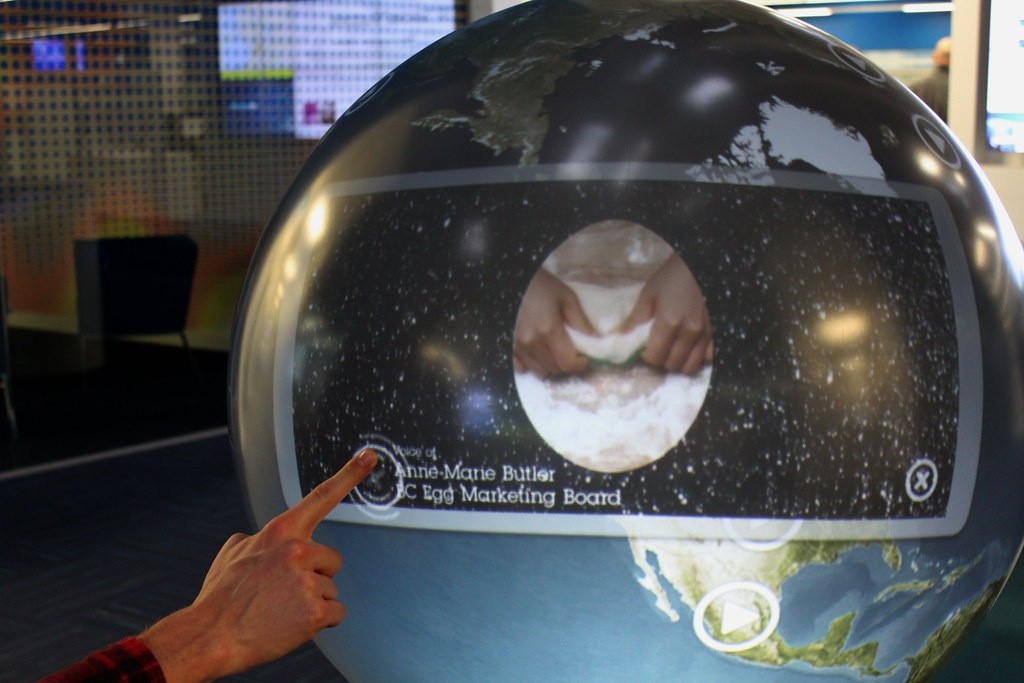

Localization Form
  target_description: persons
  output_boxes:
[907,38,950,123]
[513,220,713,376]
[36,448,377,683]
